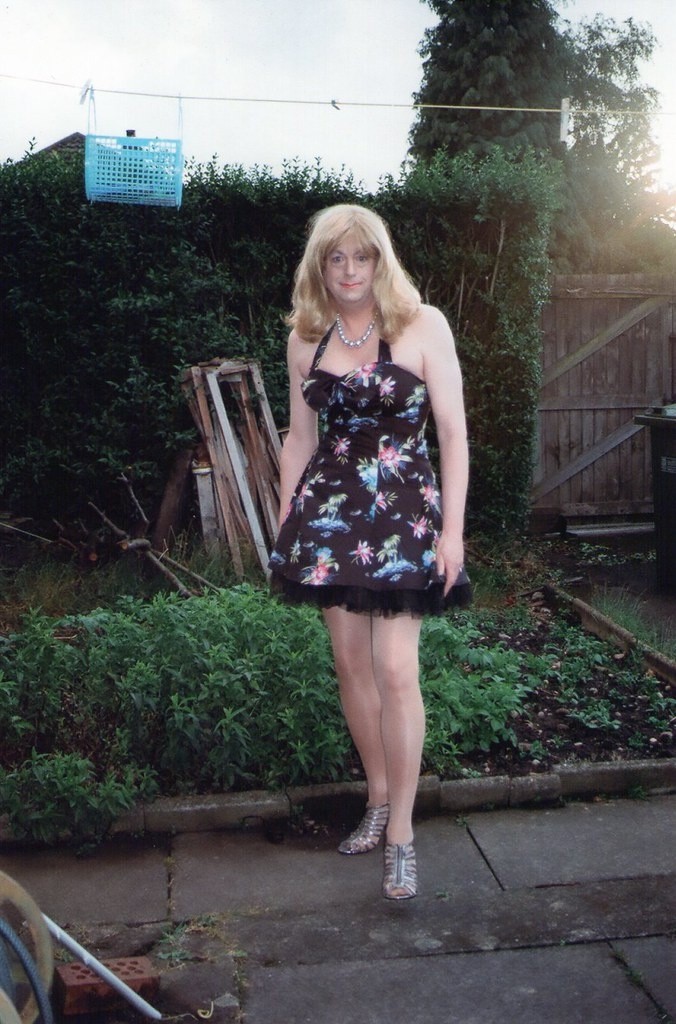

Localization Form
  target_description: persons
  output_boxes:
[266,203,470,901]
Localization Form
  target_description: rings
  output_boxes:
[458,565,463,573]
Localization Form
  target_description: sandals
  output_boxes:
[382,839,417,899]
[338,803,390,854]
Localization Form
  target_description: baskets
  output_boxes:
[85,135,184,209]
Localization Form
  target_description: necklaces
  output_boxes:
[336,308,379,348]
